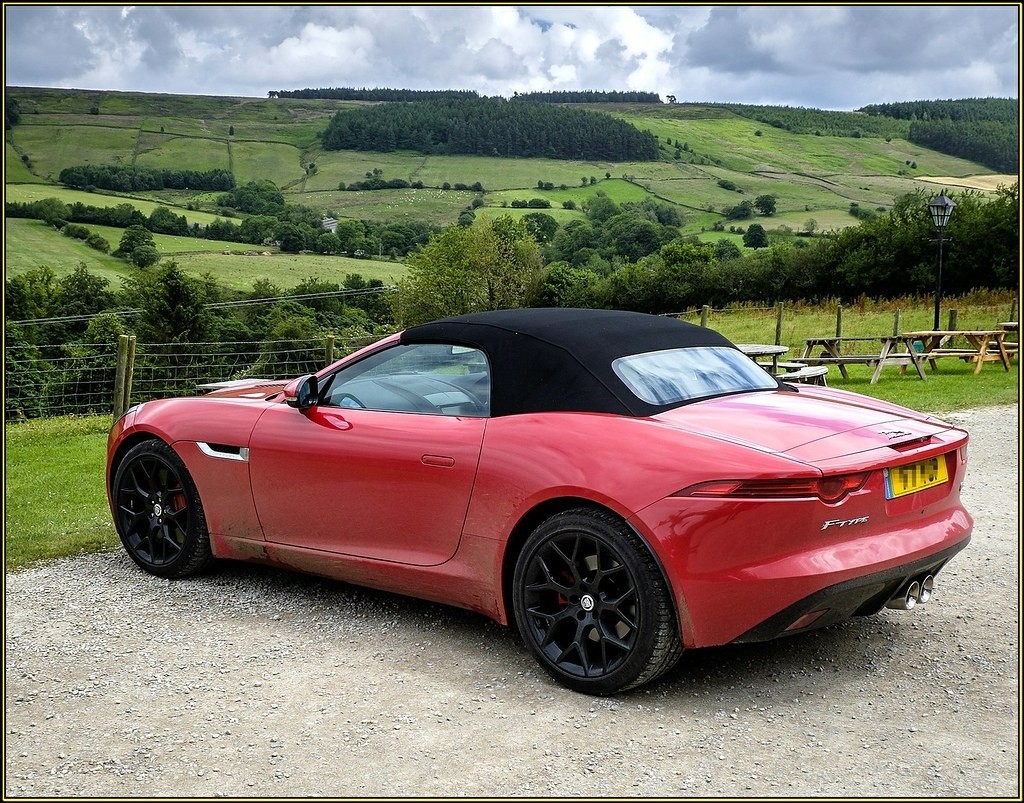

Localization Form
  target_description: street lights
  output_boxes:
[929,188,957,348]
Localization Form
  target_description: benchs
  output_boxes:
[931,349,1018,355]
[775,366,829,387]
[819,353,937,361]
[788,358,885,364]
[989,342,1019,348]
[893,352,983,357]
[758,362,809,373]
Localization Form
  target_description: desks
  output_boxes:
[196,378,271,396]
[795,335,926,385]
[991,322,1019,364]
[735,343,789,375]
[899,330,1011,375]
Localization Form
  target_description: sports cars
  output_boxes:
[104,309,974,700]
